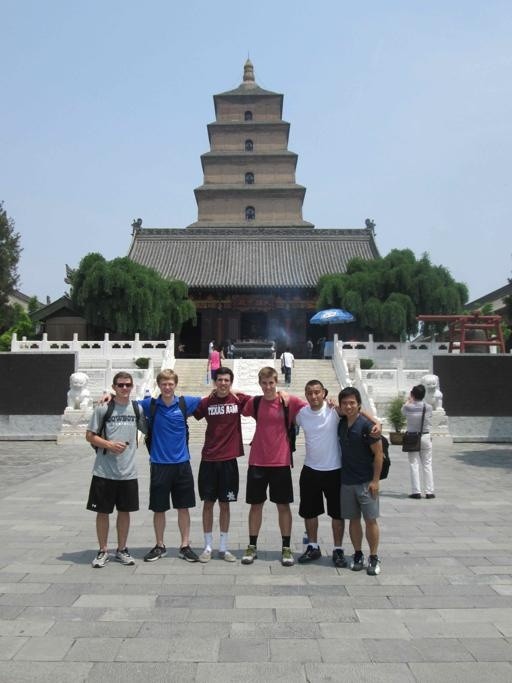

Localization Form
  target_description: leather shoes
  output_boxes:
[426,494,434,499]
[409,494,421,499]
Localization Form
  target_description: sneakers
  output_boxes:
[179,545,212,562]
[92,552,110,568]
[144,544,167,561]
[242,545,256,564]
[282,546,321,566]
[219,551,236,561]
[117,548,135,565]
[333,549,380,574]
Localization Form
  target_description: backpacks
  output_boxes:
[361,421,390,480]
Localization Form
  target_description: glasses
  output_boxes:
[118,383,132,387]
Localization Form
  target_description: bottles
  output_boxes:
[143,390,151,399]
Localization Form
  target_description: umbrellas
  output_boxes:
[310,308,356,326]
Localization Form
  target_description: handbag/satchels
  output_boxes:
[282,366,286,374]
[403,431,421,451]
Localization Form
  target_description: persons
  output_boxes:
[208,367,334,566]
[178,343,186,358]
[337,387,384,575]
[97,369,239,562]
[209,339,214,359]
[206,345,224,380]
[293,380,382,568]
[280,347,295,386]
[306,336,327,357]
[86,371,147,568]
[400,385,435,500]
[186,367,290,563]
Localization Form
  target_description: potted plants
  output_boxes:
[382,390,410,445]
